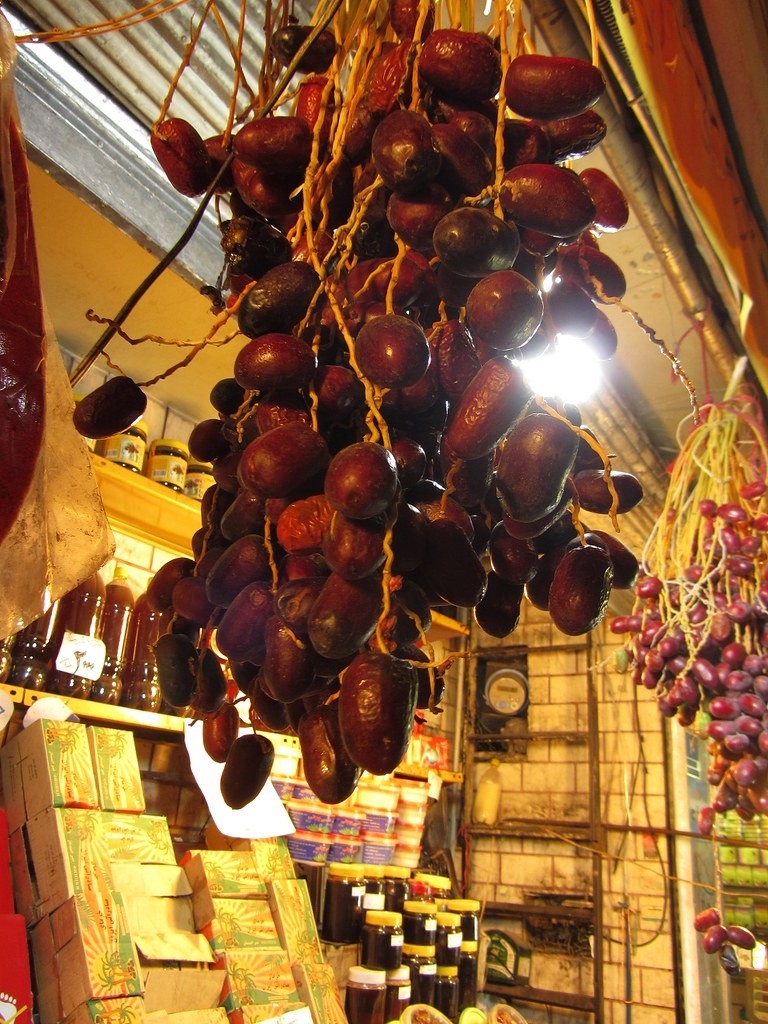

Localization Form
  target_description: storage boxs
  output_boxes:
[0,717,364,1024]
[721,847,768,930]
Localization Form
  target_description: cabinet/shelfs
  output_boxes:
[24,453,304,759]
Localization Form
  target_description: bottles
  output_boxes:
[75,395,216,501]
[0,566,196,718]
[321,862,481,1024]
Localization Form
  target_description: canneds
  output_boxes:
[103,420,149,474]
[146,438,189,492]
[182,458,216,501]
[320,862,481,1024]
[72,394,100,452]
